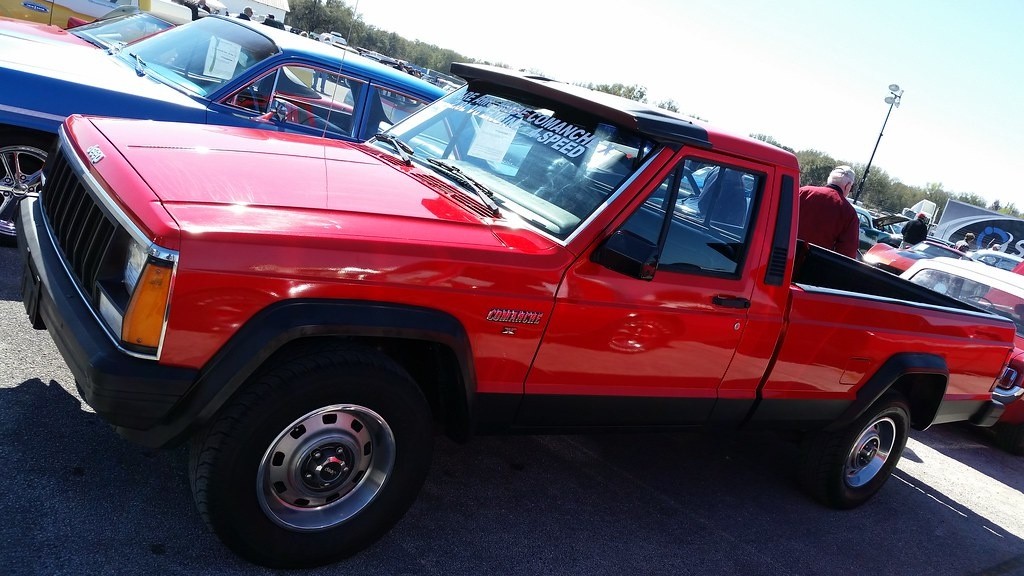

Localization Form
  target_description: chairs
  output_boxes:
[512,153,654,260]
[226,75,277,106]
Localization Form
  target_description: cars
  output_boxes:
[0,11,197,51]
[292,27,1024,460]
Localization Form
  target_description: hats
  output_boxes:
[918,213,925,218]
[834,165,855,181]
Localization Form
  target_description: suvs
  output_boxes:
[0,14,461,251]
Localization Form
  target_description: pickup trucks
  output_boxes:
[16,60,1019,573]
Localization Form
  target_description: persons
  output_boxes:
[898,214,927,251]
[697,166,746,226]
[985,244,1001,265]
[237,6,253,21]
[796,164,861,261]
[950,233,974,259]
[311,37,331,93]
[171,0,230,21]
[392,58,424,79]
[261,15,285,30]
[300,30,309,37]
